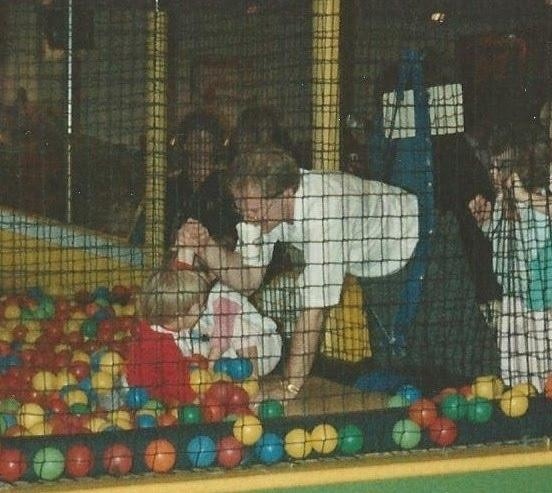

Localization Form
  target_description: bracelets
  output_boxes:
[276,376,300,396]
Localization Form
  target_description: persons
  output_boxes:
[468,101,552,394]
[128,80,305,267]
[178,142,501,416]
[136,268,282,380]
